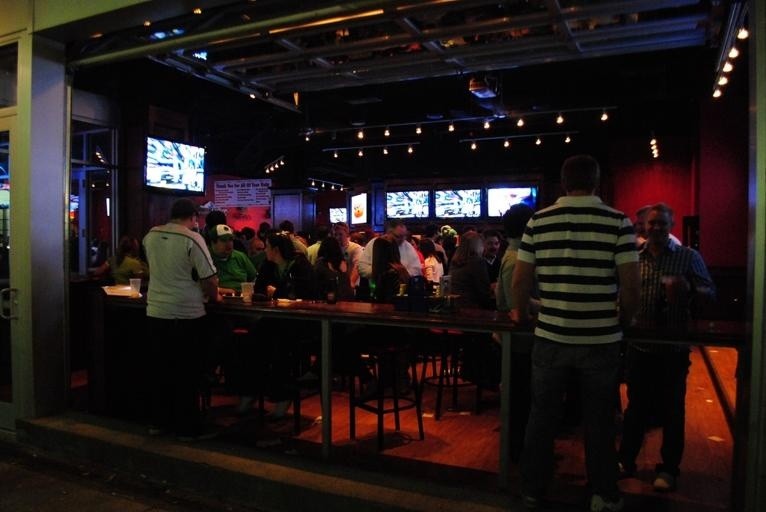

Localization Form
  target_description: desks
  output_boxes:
[104,286,746,487]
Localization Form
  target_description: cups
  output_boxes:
[239,281,254,302]
[129,278,142,298]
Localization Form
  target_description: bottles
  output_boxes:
[327,272,336,304]
[396,272,454,317]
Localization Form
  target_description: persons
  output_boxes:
[506,150,645,511]
[617,201,719,497]
[91,234,150,284]
[142,200,225,442]
[200,210,506,396]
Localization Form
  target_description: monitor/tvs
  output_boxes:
[144,135,208,196]
[433,188,485,221]
[486,186,539,223]
[387,190,430,219]
[351,193,370,225]
[330,208,347,224]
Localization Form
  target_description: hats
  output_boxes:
[208,224,235,240]
[169,198,200,218]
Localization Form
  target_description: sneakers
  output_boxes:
[240,398,256,411]
[654,477,672,490]
[591,493,623,511]
[273,400,291,417]
